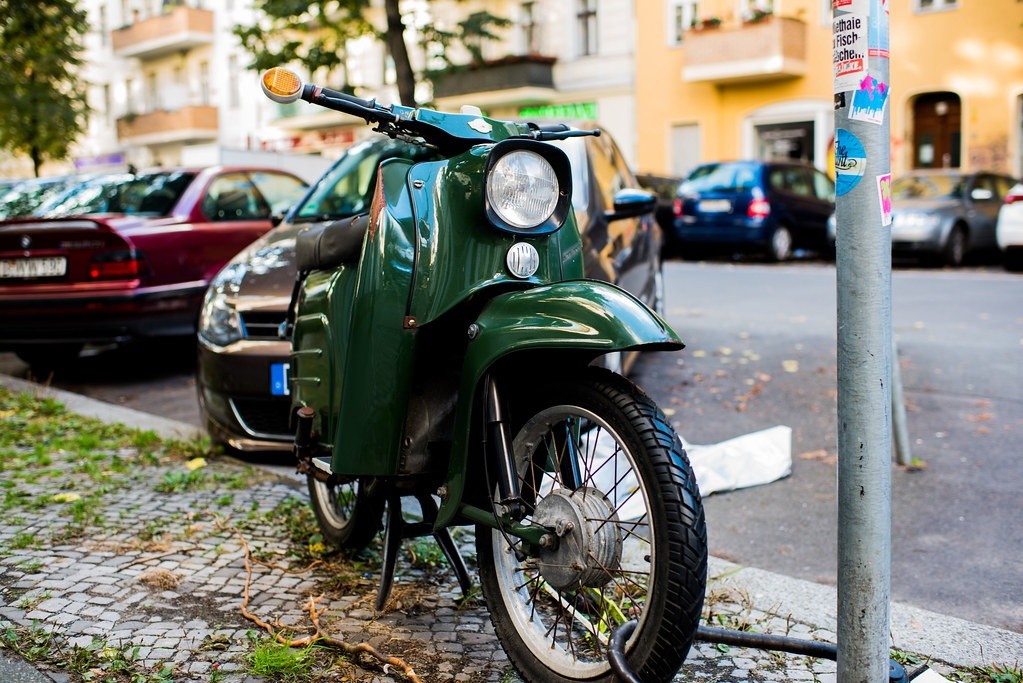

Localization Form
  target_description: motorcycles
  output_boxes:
[258,63,712,683]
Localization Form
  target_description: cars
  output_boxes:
[622,172,683,254]
[198,108,670,463]
[991,179,1022,274]
[674,159,842,260]
[0,170,138,229]
[828,166,1018,276]
[0,164,330,385]
